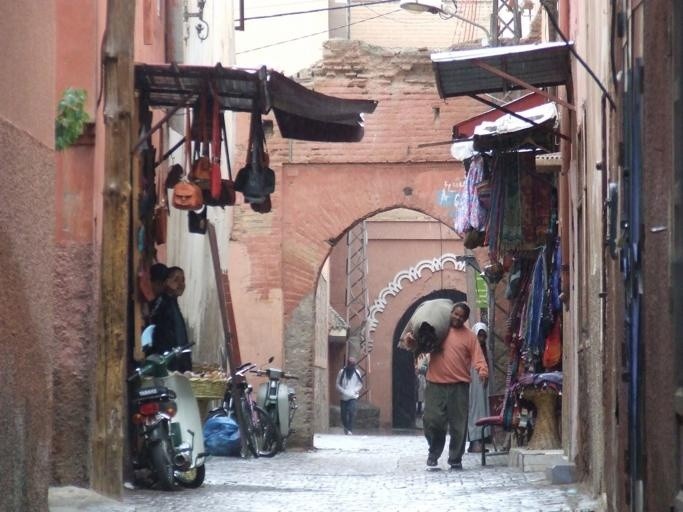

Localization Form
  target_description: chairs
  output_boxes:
[475,389,523,467]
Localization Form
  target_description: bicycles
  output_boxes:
[225,363,281,459]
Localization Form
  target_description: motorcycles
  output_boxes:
[127,342,206,490]
[250,357,298,450]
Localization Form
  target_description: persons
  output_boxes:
[414,352,431,416]
[139,265,192,375]
[142,262,168,323]
[462,322,493,453]
[404,302,489,472]
[335,356,363,436]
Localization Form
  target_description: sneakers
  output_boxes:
[467,443,490,453]
[343,429,353,435]
[426,452,438,467]
[451,461,463,469]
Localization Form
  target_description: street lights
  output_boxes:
[402,3,498,44]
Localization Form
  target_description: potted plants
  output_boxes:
[52,84,94,152]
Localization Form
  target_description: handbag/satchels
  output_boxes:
[188,207,207,234]
[165,151,275,213]
